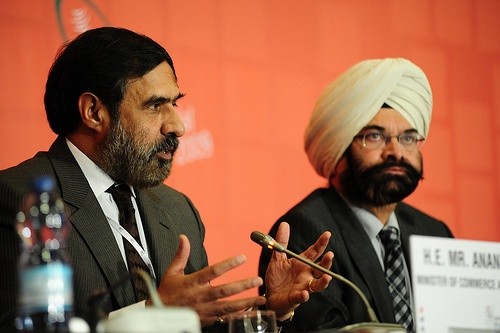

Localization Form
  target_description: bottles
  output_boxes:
[14,175,73,333]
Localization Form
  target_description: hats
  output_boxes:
[304,57,434,180]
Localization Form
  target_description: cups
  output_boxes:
[229,310,279,333]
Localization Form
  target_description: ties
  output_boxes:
[105,183,156,302]
[378,226,414,333]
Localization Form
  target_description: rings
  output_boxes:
[307,280,314,293]
[216,316,225,324]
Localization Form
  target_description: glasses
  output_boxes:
[354,128,424,151]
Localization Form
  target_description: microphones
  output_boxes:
[250,231,406,333]
[88,268,200,333]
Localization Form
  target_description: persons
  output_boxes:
[0,26,334,333]
[258,58,455,333]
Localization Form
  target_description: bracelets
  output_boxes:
[279,310,295,322]
